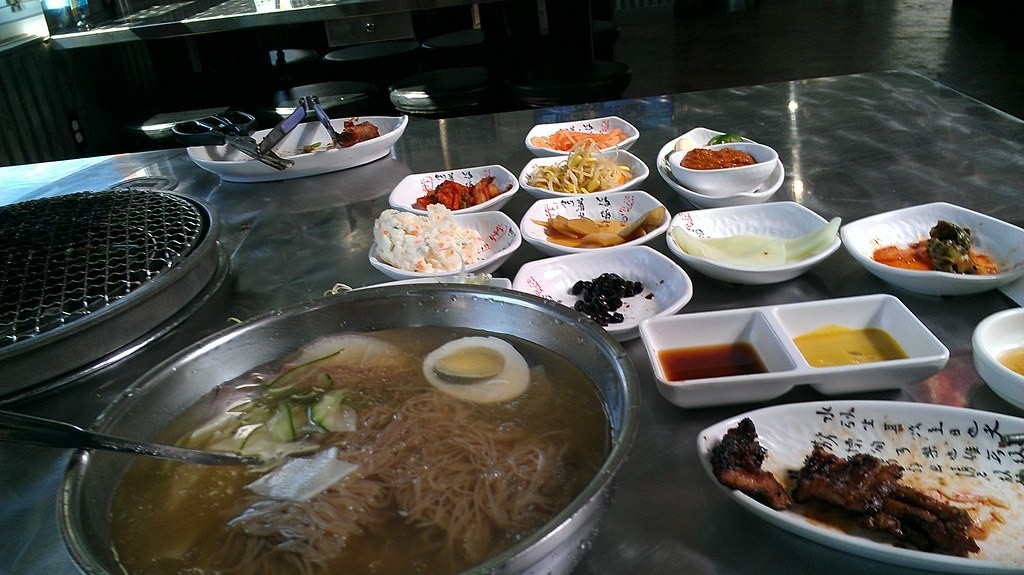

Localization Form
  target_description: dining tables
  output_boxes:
[51,0,480,52]
[0,68,1024,575]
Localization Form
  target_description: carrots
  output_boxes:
[532,128,629,151]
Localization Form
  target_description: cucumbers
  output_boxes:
[235,348,358,450]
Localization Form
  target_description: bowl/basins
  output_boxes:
[668,142,779,196]
[971,307,1024,412]
[53,282,640,575]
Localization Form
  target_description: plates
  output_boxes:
[656,127,785,207]
[638,292,950,409]
[839,201,1024,296]
[368,210,522,280]
[520,190,671,256]
[388,164,520,218]
[513,245,693,342]
[187,113,409,182]
[524,115,640,157]
[696,399,1024,575]
[664,201,841,285]
[518,149,650,199]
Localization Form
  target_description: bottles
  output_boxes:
[70,0,92,31]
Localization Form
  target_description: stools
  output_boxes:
[128,29,485,139]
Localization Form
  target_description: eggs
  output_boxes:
[422,336,530,404]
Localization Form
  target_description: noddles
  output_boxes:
[109,326,610,575]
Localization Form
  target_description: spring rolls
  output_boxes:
[340,121,380,147]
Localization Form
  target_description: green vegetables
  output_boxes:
[928,219,977,274]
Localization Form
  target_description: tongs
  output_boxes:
[257,95,343,157]
